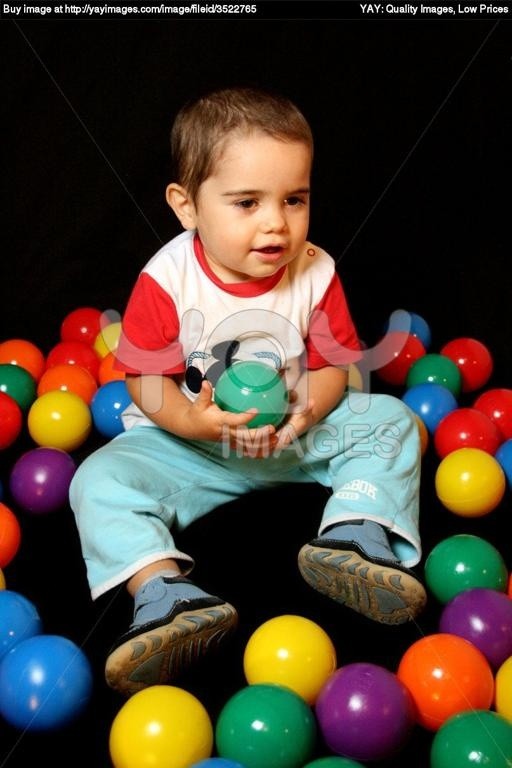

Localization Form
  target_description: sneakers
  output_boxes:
[103,575,238,694]
[298,522,428,626]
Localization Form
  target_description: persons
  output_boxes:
[66,89,429,698]
